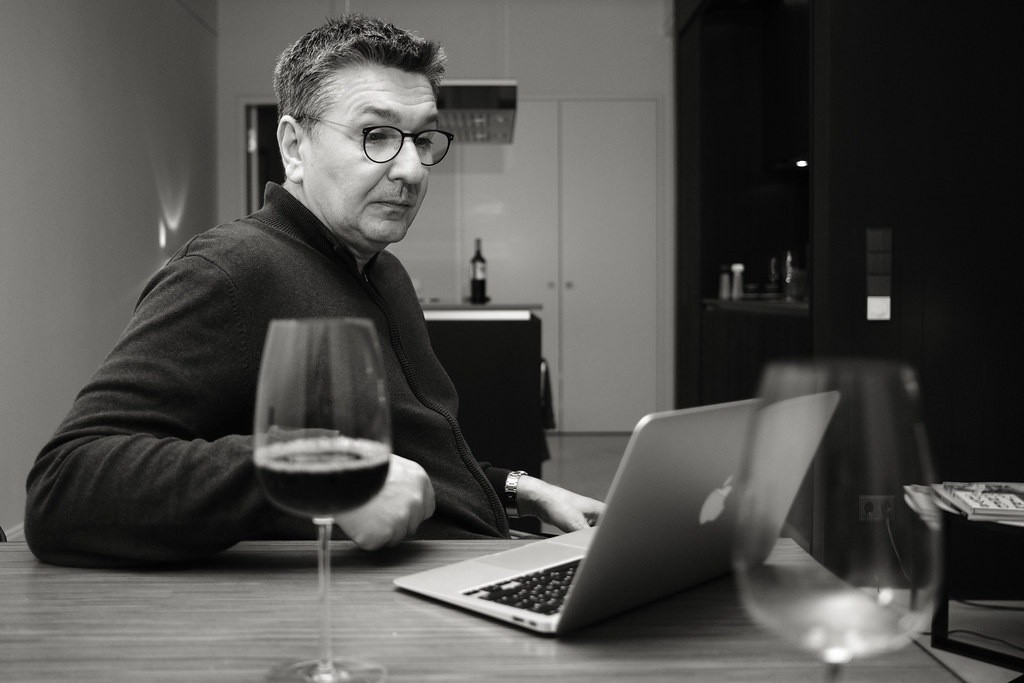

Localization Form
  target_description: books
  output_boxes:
[929,484,1024,521]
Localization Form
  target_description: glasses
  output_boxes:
[296,112,456,168]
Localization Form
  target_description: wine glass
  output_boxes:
[253,319,391,683]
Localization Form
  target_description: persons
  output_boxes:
[24,16,608,561]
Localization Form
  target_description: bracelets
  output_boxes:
[505,471,526,519]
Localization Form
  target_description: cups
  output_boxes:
[731,359,942,683]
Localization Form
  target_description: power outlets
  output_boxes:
[858,496,898,523]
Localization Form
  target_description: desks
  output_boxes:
[420,299,549,478]
[700,299,811,406]
[0,538,965,683]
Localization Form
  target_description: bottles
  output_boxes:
[718,241,804,305]
[469,238,487,305]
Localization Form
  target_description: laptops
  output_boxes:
[394,387,843,635]
